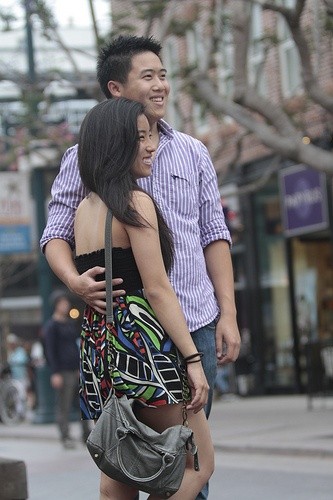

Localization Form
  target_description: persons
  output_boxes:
[0,291,95,450]
[40,36,242,500]
[73,98,215,500]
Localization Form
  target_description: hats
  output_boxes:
[49,290,71,309]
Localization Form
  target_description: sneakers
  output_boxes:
[63,437,75,450]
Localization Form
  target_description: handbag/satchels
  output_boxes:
[87,394,200,498]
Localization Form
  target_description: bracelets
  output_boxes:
[185,352,205,364]
